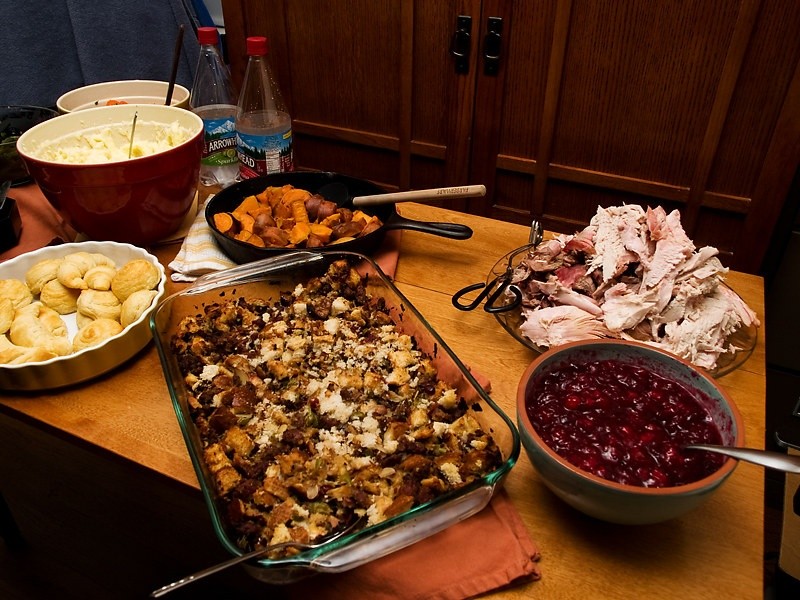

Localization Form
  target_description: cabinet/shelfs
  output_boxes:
[221,0,800,272]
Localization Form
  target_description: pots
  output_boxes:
[204,171,473,266]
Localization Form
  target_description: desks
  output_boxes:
[0,178,770,600]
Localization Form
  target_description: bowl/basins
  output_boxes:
[57,80,190,114]
[0,105,60,188]
[148,251,520,584]
[16,104,204,248]
[515,339,745,527]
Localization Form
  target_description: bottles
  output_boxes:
[234,36,294,182]
[188,27,239,189]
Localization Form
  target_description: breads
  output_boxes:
[0,250,161,365]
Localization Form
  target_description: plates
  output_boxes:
[0,240,170,390]
[487,240,758,379]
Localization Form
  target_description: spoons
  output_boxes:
[319,182,487,207]
[152,513,361,597]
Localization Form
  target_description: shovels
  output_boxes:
[315,181,485,209]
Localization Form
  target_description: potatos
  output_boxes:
[212,184,382,249]
